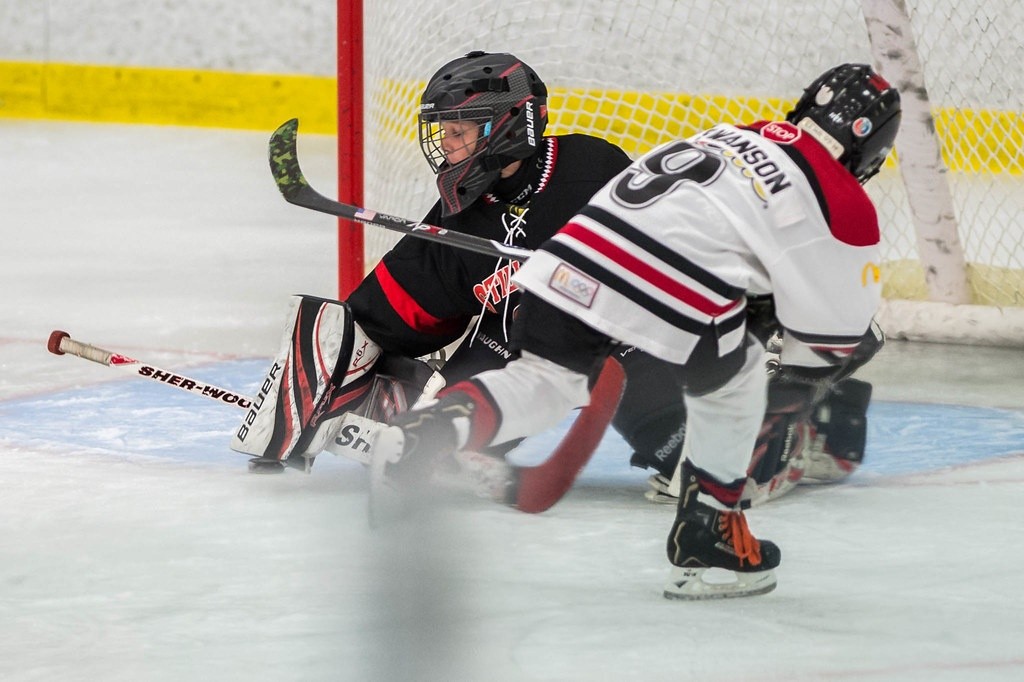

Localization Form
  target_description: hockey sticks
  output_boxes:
[266,116,537,262]
[46,327,631,517]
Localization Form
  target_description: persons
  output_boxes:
[229,51,905,602]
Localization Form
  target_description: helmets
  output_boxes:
[796,64,902,186]
[418,50,548,218]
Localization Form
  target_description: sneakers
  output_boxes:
[368,389,478,532]
[663,461,781,599]
